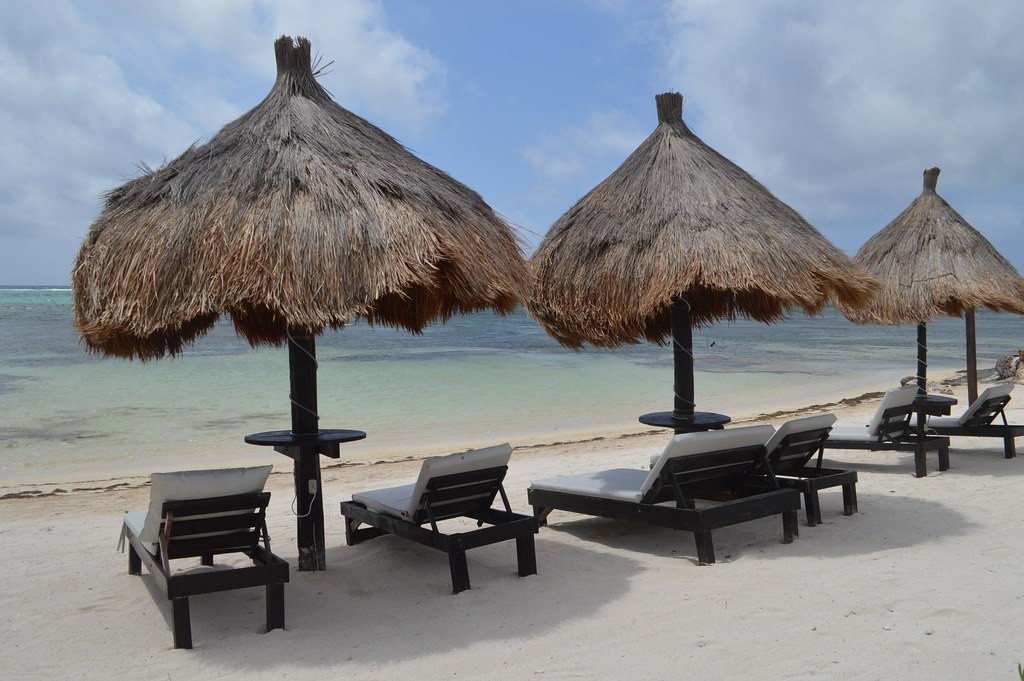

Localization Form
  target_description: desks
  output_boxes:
[913,395,957,437]
[245,429,366,572]
[639,411,731,435]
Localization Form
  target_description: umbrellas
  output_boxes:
[836,166,1024,437]
[69,31,527,572]
[526,90,878,437]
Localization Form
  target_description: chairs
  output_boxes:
[823,385,957,478]
[341,443,539,594]
[651,414,859,527]
[904,385,1024,458]
[124,465,289,650]
[527,425,801,565]
